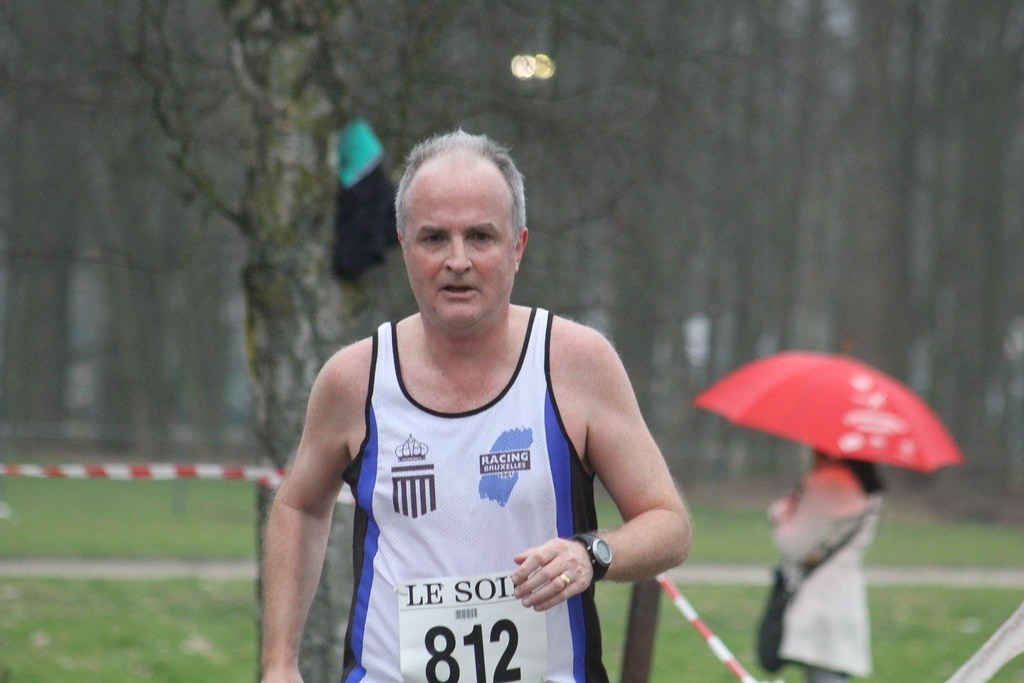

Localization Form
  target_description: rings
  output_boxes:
[561,574,574,588]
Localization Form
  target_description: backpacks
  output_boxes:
[327,116,400,280]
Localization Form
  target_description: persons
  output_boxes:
[260,127,692,683]
[766,448,887,683]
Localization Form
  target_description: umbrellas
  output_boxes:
[692,344,961,474]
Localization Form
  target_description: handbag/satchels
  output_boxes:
[757,568,794,672]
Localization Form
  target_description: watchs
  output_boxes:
[567,532,610,583]
